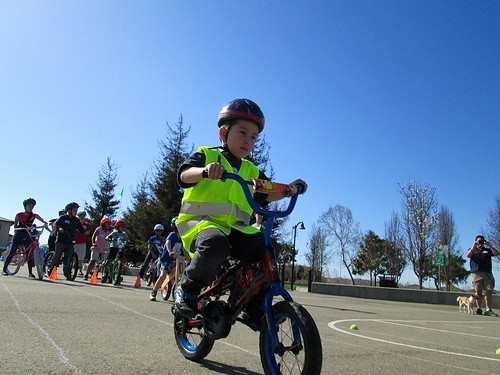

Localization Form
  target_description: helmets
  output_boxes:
[101,217,111,224]
[171,217,178,231]
[115,220,126,227]
[217,98,265,133]
[23,198,36,206]
[59,209,67,216]
[77,211,87,216]
[110,219,117,227]
[154,224,164,230]
[65,202,80,211]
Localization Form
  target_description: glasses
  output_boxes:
[155,229,163,232]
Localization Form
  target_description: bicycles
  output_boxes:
[171,170,323,375]
[83,237,130,286]
[2,223,47,281]
[43,230,84,282]
[144,250,161,286]
[160,253,188,301]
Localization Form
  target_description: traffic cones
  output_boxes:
[134,275,142,289]
[89,270,99,285]
[49,265,59,281]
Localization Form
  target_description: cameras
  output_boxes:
[479,240,486,244]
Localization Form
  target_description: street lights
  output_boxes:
[290,221,306,291]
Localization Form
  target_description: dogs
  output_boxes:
[456,295,478,314]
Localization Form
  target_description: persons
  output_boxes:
[83,216,112,280]
[252,212,265,228]
[101,220,129,282]
[2,198,52,278]
[149,217,191,301]
[175,98,308,329]
[74,210,90,275]
[137,224,166,280]
[467,235,500,317]
[45,209,67,252]
[44,202,83,277]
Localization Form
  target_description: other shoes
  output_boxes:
[150,290,157,301]
[173,284,202,318]
[47,268,51,276]
[476,308,482,315]
[83,275,88,280]
[485,309,497,317]
